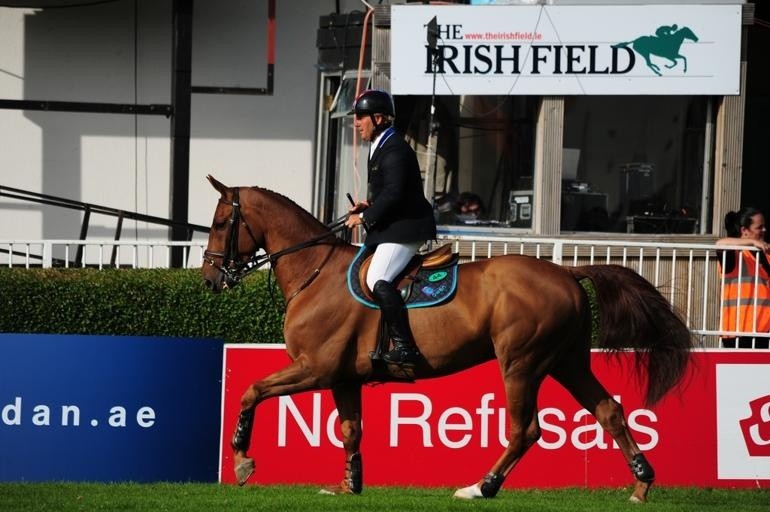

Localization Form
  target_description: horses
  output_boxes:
[201,173,710,506]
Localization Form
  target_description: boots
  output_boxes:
[373,280,416,368]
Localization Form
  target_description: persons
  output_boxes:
[454,192,499,226]
[715,207,770,348]
[571,207,608,232]
[344,91,437,368]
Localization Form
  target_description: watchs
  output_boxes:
[359,212,364,223]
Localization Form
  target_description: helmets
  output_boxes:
[348,91,393,116]
